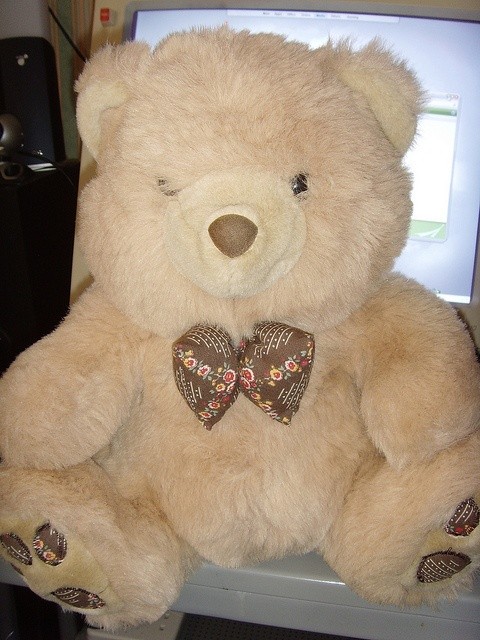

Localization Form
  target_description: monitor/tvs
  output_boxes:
[69,0,478,353]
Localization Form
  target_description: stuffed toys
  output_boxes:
[0,26,478,631]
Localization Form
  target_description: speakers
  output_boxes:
[1,36,67,173]
